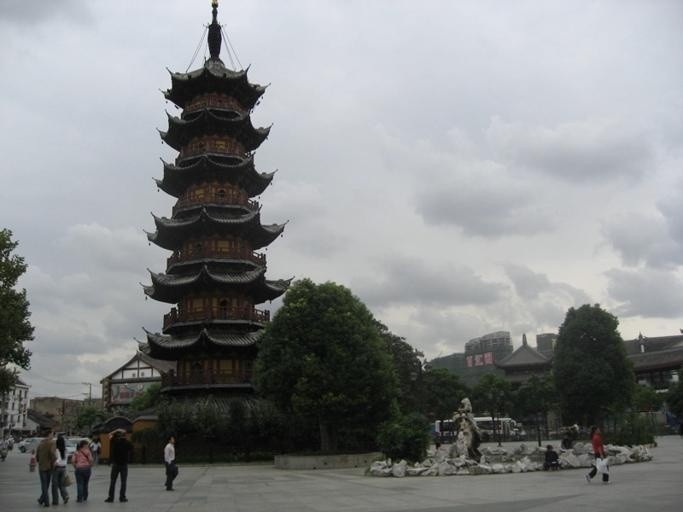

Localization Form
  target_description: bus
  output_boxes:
[434,418,524,443]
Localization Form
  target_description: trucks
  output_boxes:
[638,410,677,425]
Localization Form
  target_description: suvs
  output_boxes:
[548,426,575,439]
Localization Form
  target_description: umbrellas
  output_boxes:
[431,431,441,435]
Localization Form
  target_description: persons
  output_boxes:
[0,437,9,457]
[52,437,69,504]
[8,435,14,444]
[164,436,178,490]
[90,439,101,461]
[543,428,577,470]
[586,427,611,484]
[71,440,93,502]
[35,430,54,506]
[434,435,441,450]
[104,429,134,502]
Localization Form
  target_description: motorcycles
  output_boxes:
[8,440,15,449]
[1,447,9,461]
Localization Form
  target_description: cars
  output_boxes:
[20,436,99,466]
